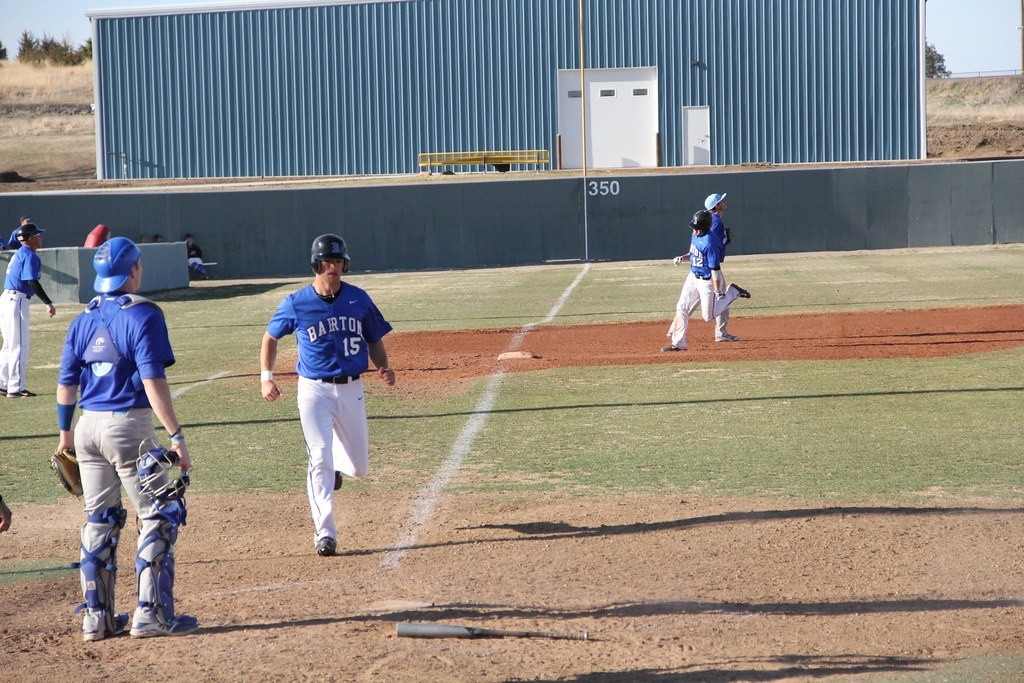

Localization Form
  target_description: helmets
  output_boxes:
[311,234,350,272]
[135,435,193,510]
[93,237,141,293]
[705,193,727,210]
[688,211,713,231]
[17,223,45,241]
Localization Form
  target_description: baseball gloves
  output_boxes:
[46,448,83,502]
[726,227,733,245]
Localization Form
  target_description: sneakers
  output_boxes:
[130,610,198,638]
[83,612,128,641]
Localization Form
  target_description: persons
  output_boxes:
[6,215,33,249]
[140,234,148,242]
[260,233,395,556]
[184,233,209,280]
[0,234,6,250]
[666,193,740,342]
[0,224,56,399]
[661,209,751,351]
[0,494,12,533]
[154,234,164,243]
[55,237,199,640]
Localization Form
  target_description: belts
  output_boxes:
[695,276,711,280]
[315,375,359,384]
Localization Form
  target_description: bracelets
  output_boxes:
[261,371,273,382]
[168,428,185,443]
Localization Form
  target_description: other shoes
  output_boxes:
[334,471,342,490]
[715,335,739,342]
[317,538,336,555]
[730,283,750,298]
[661,345,679,351]
[0,388,36,398]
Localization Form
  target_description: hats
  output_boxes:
[20,215,32,222]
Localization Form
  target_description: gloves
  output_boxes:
[673,256,683,266]
[716,291,726,299]
